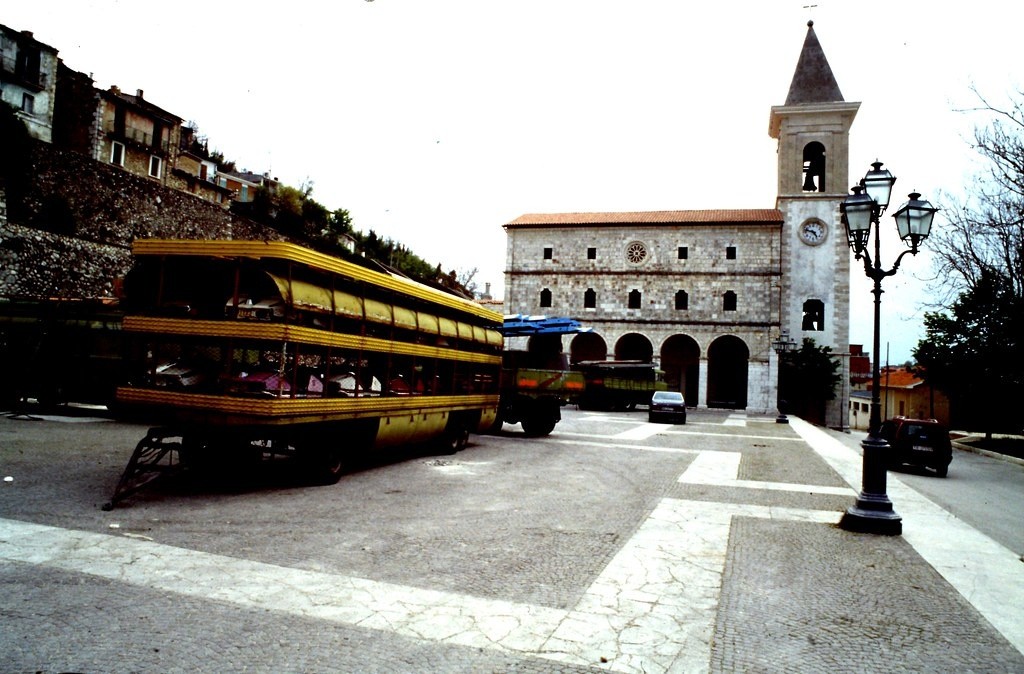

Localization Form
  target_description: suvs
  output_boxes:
[865,412,954,478]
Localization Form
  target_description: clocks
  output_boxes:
[797,218,828,245]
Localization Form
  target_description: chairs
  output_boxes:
[181,294,501,406]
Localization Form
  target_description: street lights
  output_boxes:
[838,159,937,539]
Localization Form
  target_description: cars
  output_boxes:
[659,390,688,425]
